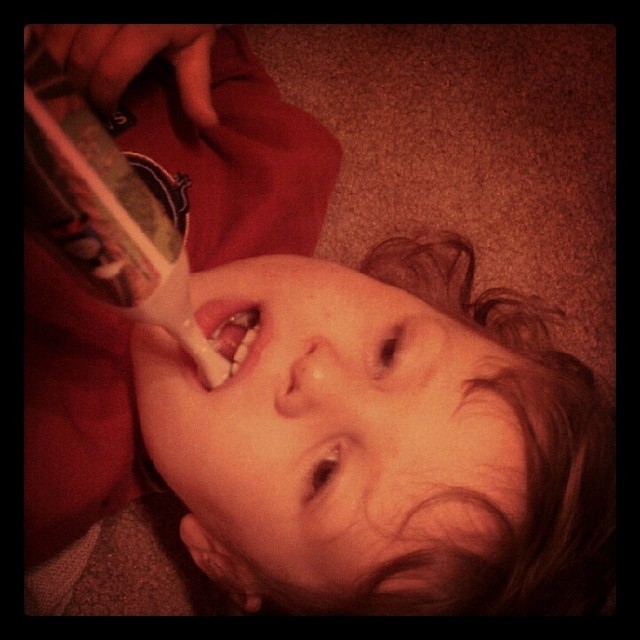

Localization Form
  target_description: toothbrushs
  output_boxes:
[23,24,232,389]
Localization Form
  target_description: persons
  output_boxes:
[22,23,615,616]
